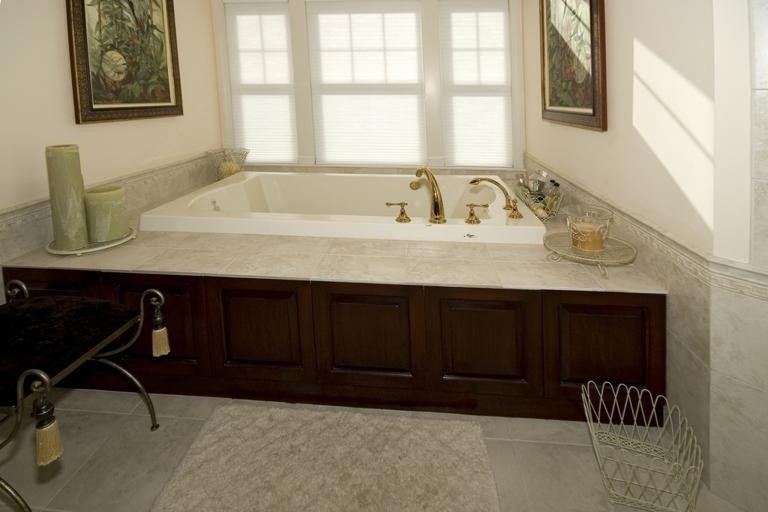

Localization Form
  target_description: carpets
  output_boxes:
[147,401,501,512]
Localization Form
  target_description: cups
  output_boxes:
[563,203,614,253]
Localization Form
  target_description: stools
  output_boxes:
[0,276,176,511]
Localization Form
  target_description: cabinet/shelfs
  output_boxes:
[546,291,666,425]
[426,286,544,419]
[206,277,315,403]
[1,271,204,397]
[315,282,424,411]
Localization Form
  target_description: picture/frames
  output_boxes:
[536,0,610,134]
[64,2,185,126]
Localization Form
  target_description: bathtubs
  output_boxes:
[140,171,549,245]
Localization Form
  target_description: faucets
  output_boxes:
[469,177,512,210]
[415,168,445,225]
